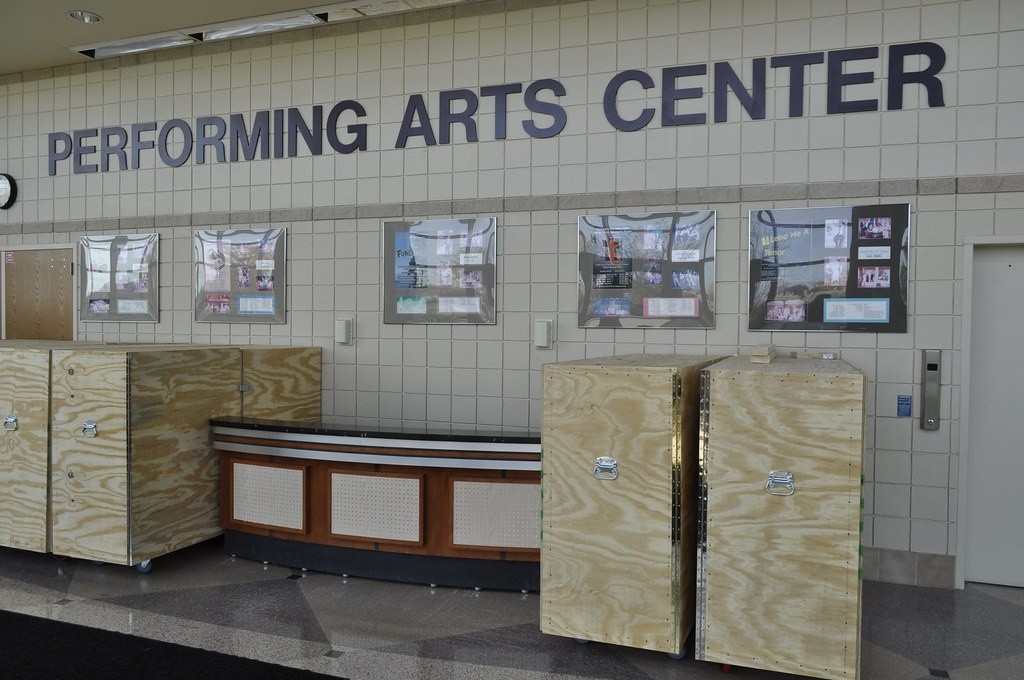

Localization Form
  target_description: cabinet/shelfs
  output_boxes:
[541,354,865,680]
[1,340,326,569]
